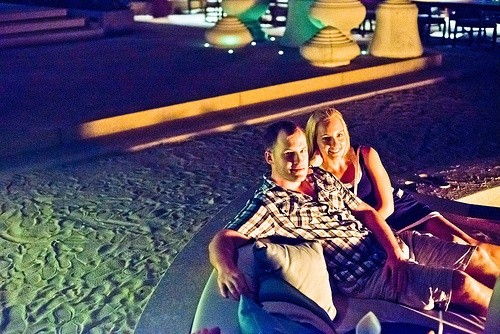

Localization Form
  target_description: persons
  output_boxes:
[305,106,500,268]
[208,120,500,318]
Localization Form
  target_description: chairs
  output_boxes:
[448,5,497,42]
[418,5,446,40]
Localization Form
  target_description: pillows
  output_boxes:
[238,293,321,334]
[252,240,337,322]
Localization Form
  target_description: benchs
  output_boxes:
[133,179,500,334]
